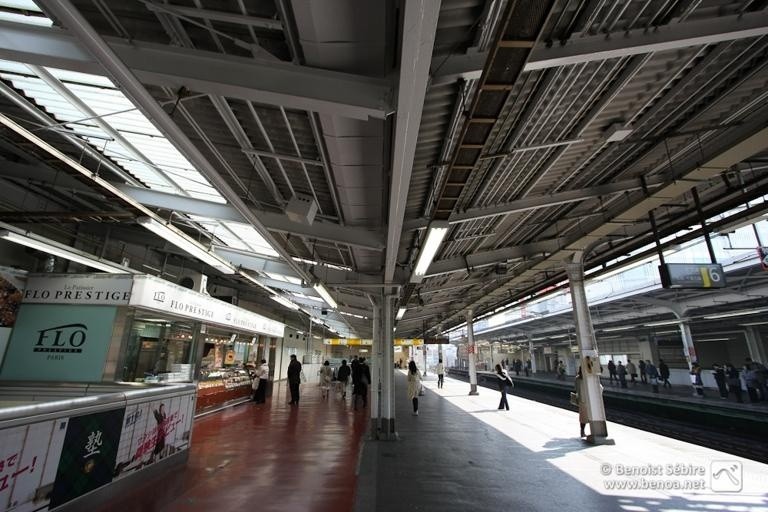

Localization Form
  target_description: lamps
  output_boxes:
[395,305,407,320]
[409,221,451,285]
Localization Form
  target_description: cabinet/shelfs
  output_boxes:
[196,367,255,414]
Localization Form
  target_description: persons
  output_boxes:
[287,354,301,404]
[557,360,564,380]
[255,359,270,404]
[689,357,767,404]
[320,355,371,411]
[408,360,422,416]
[436,359,444,389]
[149,403,166,463]
[495,364,514,410]
[502,357,531,377]
[600,360,671,393]
[575,366,604,437]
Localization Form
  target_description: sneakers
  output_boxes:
[412,410,419,416]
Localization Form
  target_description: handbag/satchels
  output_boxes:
[569,391,580,407]
[251,377,261,390]
[325,375,331,382]
[418,385,427,396]
[346,375,351,386]
